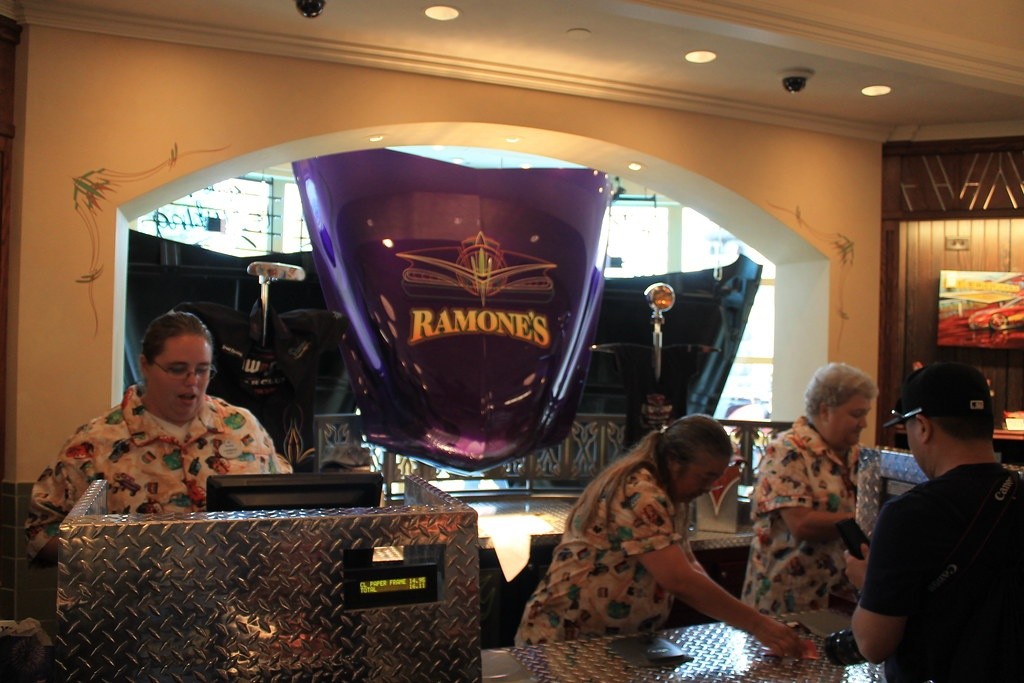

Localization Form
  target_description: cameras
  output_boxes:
[823,629,868,666]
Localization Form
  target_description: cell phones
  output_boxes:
[836,517,870,559]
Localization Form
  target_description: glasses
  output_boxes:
[143,356,217,379]
[675,459,724,491]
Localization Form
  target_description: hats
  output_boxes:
[882,361,991,428]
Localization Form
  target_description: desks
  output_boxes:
[465,498,756,648]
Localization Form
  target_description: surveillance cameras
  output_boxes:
[295,0,325,18]
[778,70,814,94]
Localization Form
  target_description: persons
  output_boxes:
[25,311,293,567]
[515,414,808,660]
[741,363,875,611]
[844,363,1024,683]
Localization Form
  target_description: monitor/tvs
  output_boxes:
[206,471,382,510]
